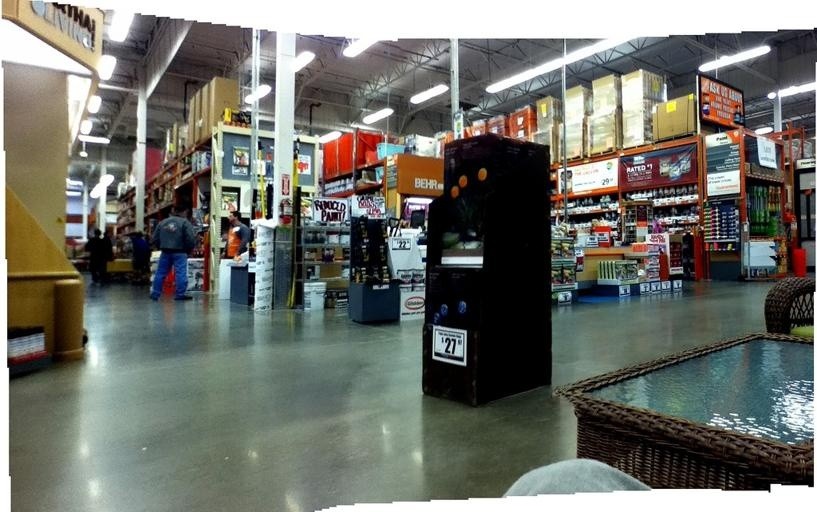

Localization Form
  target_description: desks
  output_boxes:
[551,328,813,489]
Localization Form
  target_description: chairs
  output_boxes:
[759,276,815,337]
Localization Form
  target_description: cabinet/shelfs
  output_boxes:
[112,184,139,258]
[548,157,620,241]
[293,224,349,314]
[551,233,579,305]
[348,208,400,325]
[614,134,701,247]
[576,242,631,294]
[145,140,214,255]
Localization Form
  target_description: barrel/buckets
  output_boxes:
[302,281,328,310]
[592,226,611,247]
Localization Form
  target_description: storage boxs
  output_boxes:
[434,65,696,164]
[318,129,437,177]
[160,122,188,165]
[186,75,245,148]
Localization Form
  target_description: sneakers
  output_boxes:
[150,296,158,300]
[176,295,192,300]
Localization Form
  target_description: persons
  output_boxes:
[149,204,198,300]
[222,210,252,263]
[85,229,106,287]
[137,227,150,271]
[233,242,258,262]
[101,232,114,260]
